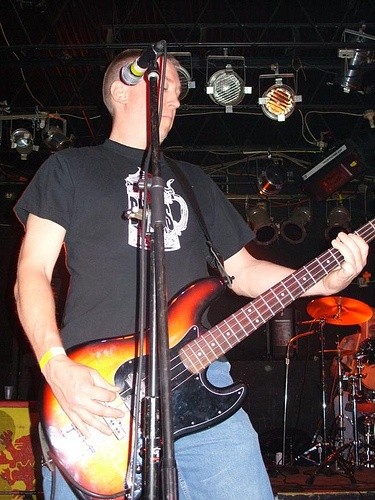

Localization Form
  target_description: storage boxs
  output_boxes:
[0,400,45,500]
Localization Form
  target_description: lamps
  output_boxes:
[166,52,195,100]
[257,157,287,196]
[325,198,353,243]
[245,196,280,246]
[327,25,375,95]
[10,116,40,161]
[281,198,314,242]
[259,63,302,122]
[42,118,73,153]
[206,47,252,114]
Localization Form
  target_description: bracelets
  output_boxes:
[39,347,66,369]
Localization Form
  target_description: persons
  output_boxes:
[13,49,368,500]
[330,305,375,474]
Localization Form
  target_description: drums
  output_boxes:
[356,336,375,391]
[348,372,374,416]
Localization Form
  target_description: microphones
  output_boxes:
[121,39,165,86]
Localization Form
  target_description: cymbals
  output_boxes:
[318,349,356,356]
[306,296,373,326]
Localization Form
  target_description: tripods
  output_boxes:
[285,318,375,486]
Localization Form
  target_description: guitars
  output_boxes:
[39,217,374,500]
